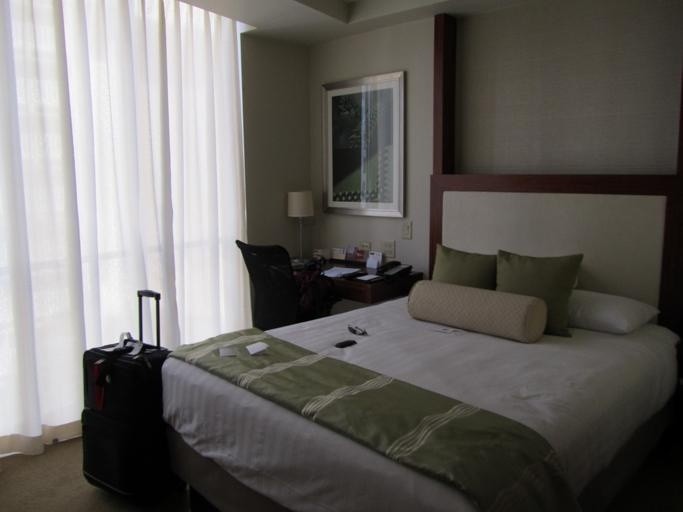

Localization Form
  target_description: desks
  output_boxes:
[314,257,424,307]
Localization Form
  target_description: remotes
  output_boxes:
[348,321,367,335]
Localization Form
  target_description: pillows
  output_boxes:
[409,242,661,344]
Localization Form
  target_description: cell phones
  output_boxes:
[334,340,357,348]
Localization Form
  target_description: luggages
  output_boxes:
[82,290,169,500]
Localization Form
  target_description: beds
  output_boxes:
[153,176,682,510]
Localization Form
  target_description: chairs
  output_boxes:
[235,239,333,331]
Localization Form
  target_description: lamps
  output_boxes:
[287,190,316,269]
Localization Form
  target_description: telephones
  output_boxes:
[375,261,412,277]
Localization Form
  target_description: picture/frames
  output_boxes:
[320,69,406,219]
